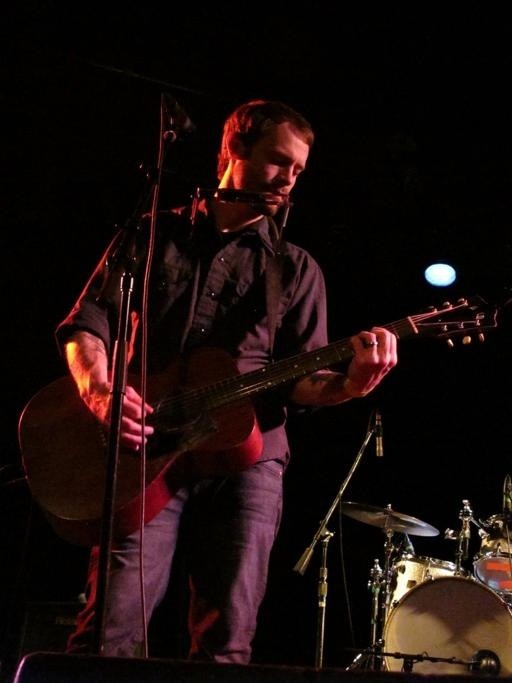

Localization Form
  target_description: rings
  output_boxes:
[364,341,378,347]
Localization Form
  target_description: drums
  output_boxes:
[473,513,512,595]
[383,556,463,630]
[381,576,512,683]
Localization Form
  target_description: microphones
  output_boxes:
[376,413,384,457]
[163,93,197,136]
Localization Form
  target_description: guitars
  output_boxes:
[14,293,504,544]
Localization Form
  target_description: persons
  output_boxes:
[54,98,399,665]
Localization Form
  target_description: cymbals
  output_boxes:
[333,494,440,541]
